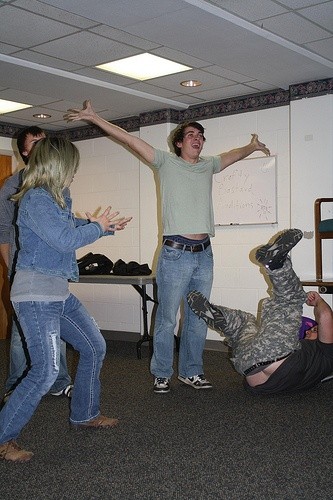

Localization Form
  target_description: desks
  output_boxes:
[68,274,155,360]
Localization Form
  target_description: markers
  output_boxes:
[230,223,239,226]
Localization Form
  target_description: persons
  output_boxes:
[0,125,75,403]
[186,228,333,396]
[67,99,271,392]
[0,137,134,464]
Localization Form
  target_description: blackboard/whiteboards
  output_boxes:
[212,155,278,226]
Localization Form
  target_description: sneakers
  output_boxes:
[48,384,74,397]
[69,414,118,428]
[186,289,227,330]
[152,376,172,393]
[0,439,34,463]
[177,374,214,389]
[255,228,303,265]
[3,390,14,405]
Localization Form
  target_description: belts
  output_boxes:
[163,238,211,252]
